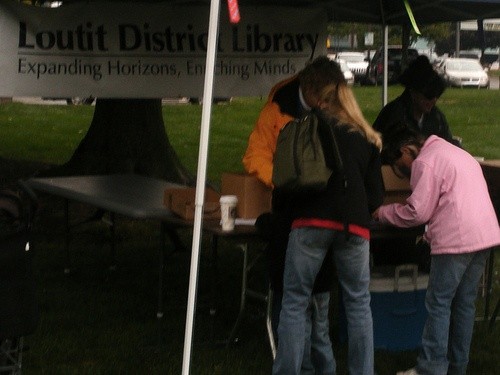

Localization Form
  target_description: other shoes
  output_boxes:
[396,365,420,375]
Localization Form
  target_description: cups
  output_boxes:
[219,195,239,231]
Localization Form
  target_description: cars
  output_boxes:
[326,48,489,88]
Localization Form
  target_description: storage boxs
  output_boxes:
[338,264,429,351]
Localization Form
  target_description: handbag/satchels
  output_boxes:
[274,113,342,187]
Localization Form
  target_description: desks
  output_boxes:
[28,174,228,349]
[160,191,426,364]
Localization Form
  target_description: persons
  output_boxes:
[372,127,500,375]
[242,56,384,375]
[372,56,453,167]
